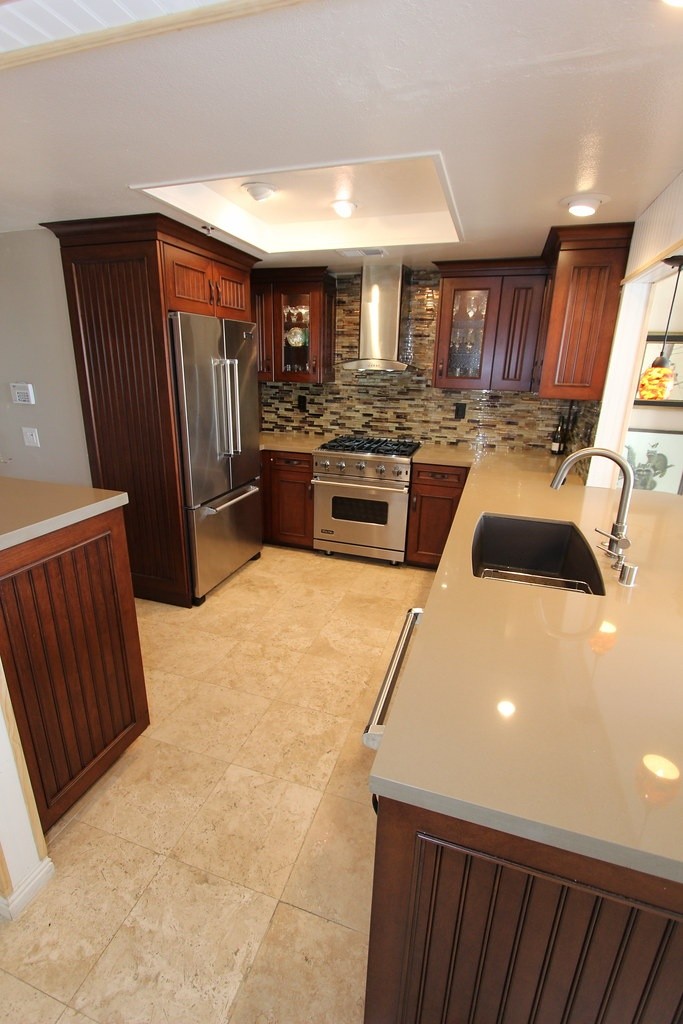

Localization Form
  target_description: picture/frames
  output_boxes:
[635,332,683,407]
[616,427,683,496]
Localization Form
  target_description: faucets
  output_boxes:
[549,447,635,558]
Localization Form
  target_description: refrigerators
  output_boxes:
[167,310,264,608]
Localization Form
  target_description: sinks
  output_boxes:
[472,510,606,598]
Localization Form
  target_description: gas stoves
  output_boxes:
[312,433,421,482]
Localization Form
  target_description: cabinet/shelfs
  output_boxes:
[0,506,152,832]
[366,799,683,1024]
[248,265,337,384]
[36,208,262,322]
[407,463,467,569]
[261,453,314,546]
[428,255,545,392]
[532,220,636,401]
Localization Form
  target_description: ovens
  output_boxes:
[311,473,409,566]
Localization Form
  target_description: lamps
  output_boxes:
[565,198,603,217]
[328,196,358,219]
[242,180,279,204]
[639,255,682,402]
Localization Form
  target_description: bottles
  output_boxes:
[551,416,566,454]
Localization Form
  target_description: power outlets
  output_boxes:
[455,402,467,419]
[297,394,307,411]
[23,425,40,450]
